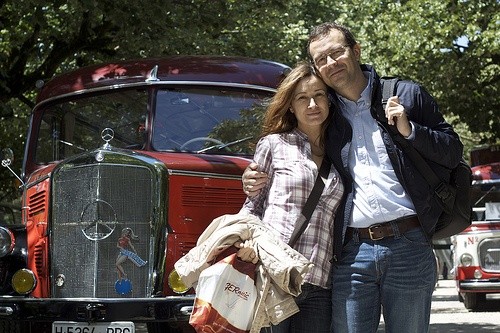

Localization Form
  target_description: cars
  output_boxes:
[447,162,500,309]
[0,55,294,333]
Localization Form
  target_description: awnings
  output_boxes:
[470,163,500,185]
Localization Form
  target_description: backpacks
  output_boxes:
[375,76,472,240]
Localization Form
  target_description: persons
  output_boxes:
[242,22,465,333]
[235,63,345,333]
[124,113,147,151]
[441,262,449,280]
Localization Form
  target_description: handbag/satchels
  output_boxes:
[188,245,257,332]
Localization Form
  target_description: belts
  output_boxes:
[344,216,421,240]
